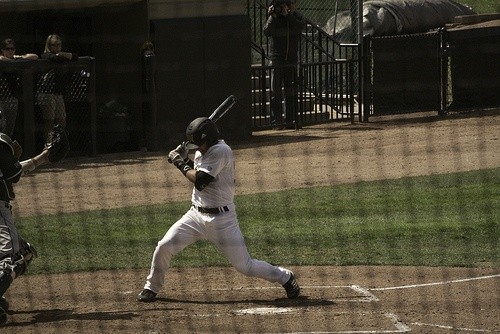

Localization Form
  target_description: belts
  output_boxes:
[192,205,229,214]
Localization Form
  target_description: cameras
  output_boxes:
[273,5,283,14]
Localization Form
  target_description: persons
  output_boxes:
[0,108,64,327]
[135,117,300,303]
[263,0,306,129]
[0,38,39,140]
[35,34,79,144]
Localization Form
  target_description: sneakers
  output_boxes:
[282,270,300,300]
[136,289,155,302]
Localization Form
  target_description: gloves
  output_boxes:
[169,151,185,167]
[176,142,188,159]
[43,124,63,152]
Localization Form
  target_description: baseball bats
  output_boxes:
[166,94,236,164]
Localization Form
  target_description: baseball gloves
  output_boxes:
[42,123,71,166]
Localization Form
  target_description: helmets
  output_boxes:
[186,117,220,144]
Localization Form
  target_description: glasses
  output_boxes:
[4,46,16,50]
